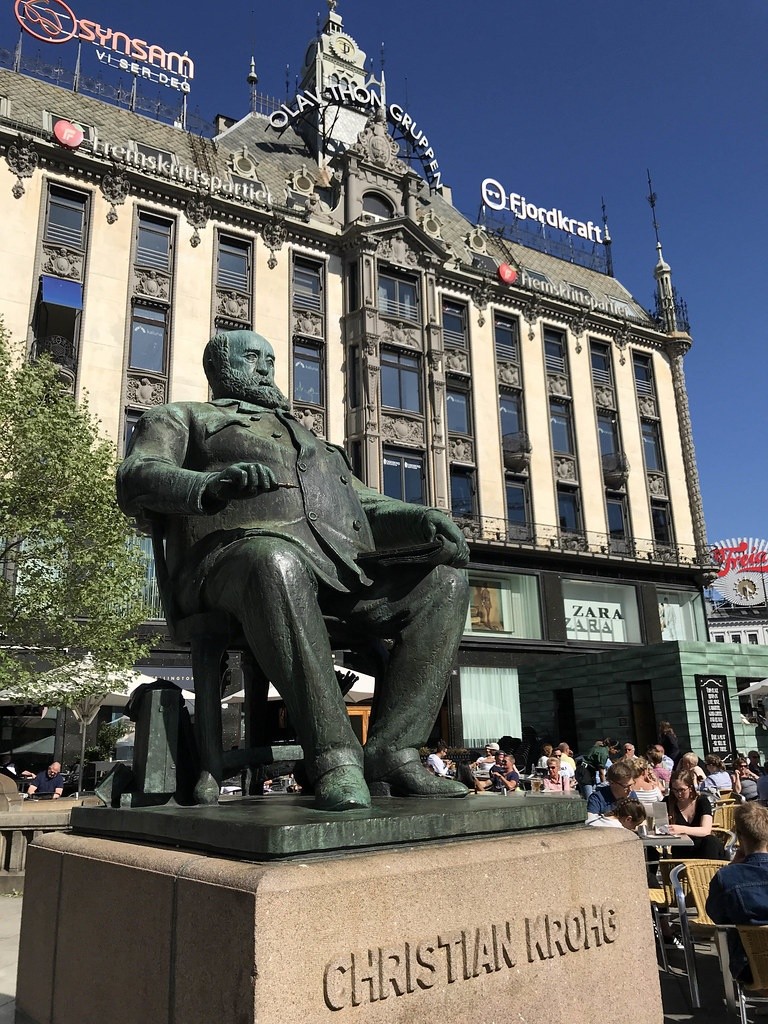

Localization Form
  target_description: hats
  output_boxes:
[486,744,500,750]
[492,750,505,756]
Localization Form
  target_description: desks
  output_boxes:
[636,830,694,857]
[475,770,492,778]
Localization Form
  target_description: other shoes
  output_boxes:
[659,931,685,950]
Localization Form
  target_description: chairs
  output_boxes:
[652,789,768,1024]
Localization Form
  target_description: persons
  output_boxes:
[658,720,679,759]
[585,798,647,830]
[0,755,64,799]
[705,801,768,986]
[115,328,471,810]
[662,769,722,860]
[426,737,768,806]
[587,762,662,889]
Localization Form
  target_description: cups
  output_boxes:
[638,825,648,838]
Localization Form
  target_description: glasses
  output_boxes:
[671,787,690,793]
[547,766,557,770]
[616,779,636,791]
[554,755,561,758]
[739,765,748,768]
[752,756,760,759]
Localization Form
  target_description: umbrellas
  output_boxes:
[0,650,195,794]
[220,665,375,704]
[730,678,768,699]
